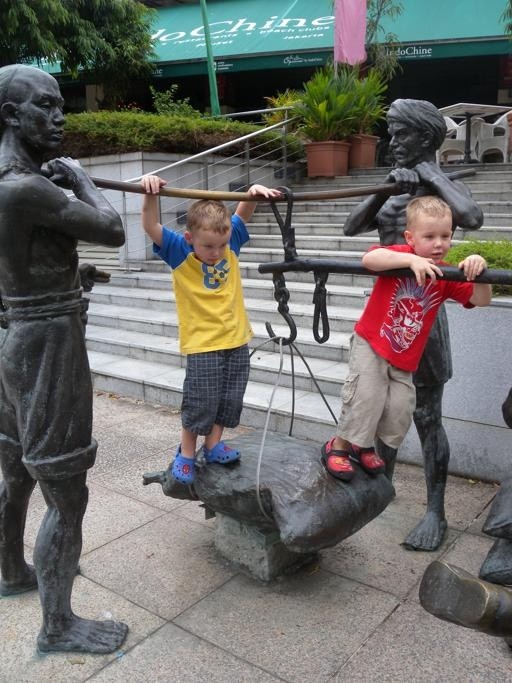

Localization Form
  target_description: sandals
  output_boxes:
[320,435,357,481]
[202,440,243,467]
[347,441,386,475]
[172,442,196,487]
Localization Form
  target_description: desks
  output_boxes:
[438,101,511,163]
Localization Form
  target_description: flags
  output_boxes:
[335,1,368,66]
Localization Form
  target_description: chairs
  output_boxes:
[436,112,509,164]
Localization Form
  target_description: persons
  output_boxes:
[1,60,131,657]
[318,190,494,482]
[138,170,284,488]
[338,96,484,552]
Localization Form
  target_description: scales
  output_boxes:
[252,186,510,344]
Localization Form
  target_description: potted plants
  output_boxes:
[281,63,389,178]
